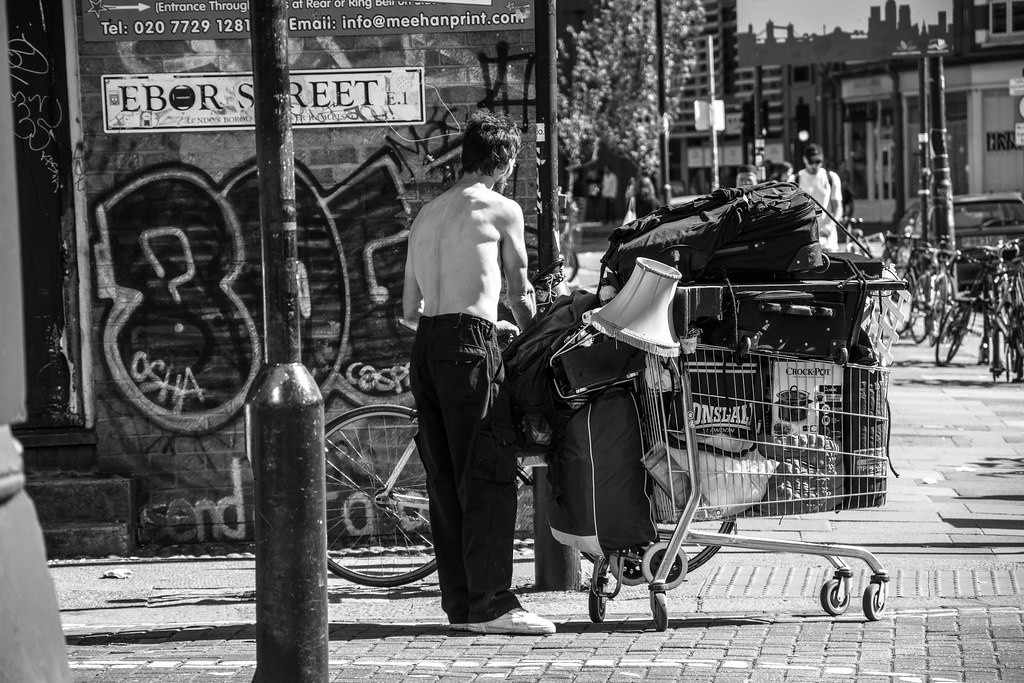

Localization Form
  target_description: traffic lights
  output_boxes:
[795,104,812,151]
[740,99,756,143]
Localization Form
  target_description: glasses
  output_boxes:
[809,159,821,165]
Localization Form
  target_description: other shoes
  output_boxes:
[449,622,468,631]
[468,607,556,634]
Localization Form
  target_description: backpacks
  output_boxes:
[545,330,645,400]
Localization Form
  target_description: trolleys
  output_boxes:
[588,351,892,635]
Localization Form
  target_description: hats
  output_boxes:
[804,144,824,162]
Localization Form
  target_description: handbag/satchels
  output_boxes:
[661,422,780,517]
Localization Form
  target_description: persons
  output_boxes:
[734,166,756,188]
[402,115,557,634]
[766,160,794,182]
[787,143,843,251]
[573,173,587,222]
[621,171,656,225]
[601,164,618,226]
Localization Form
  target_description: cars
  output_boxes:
[901,193,1024,291]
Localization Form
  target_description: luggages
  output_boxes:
[690,294,849,366]
[612,181,824,290]
[546,381,688,591]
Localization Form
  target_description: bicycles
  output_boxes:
[843,219,1024,384]
[324,326,543,588]
[557,191,581,282]
